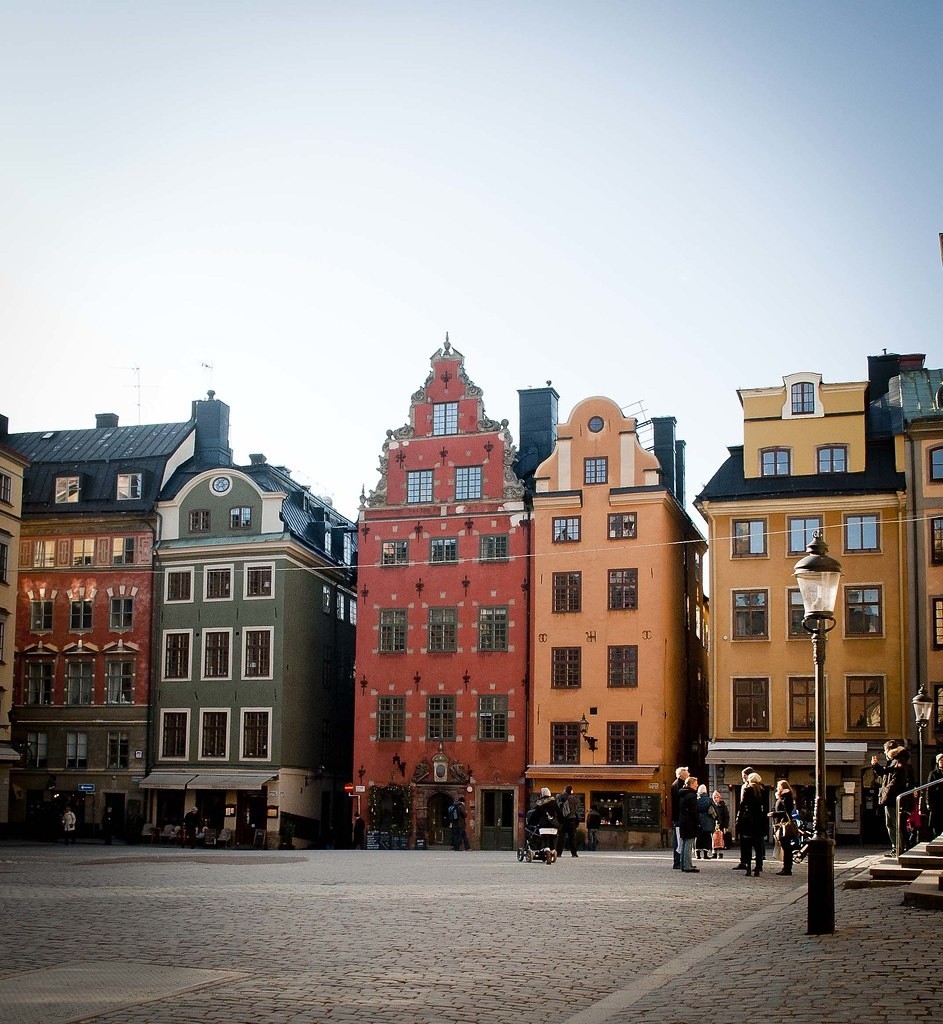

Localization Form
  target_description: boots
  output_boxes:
[544,848,557,865]
[696,851,701,859]
[703,849,711,859]
[711,854,717,859]
[718,854,723,859]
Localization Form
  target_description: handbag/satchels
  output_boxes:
[773,813,799,842]
[675,827,684,854]
[694,824,703,837]
[549,816,563,831]
[707,798,718,819]
[712,829,724,849]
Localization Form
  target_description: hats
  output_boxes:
[936,754,943,763]
[893,749,910,761]
[748,773,762,782]
[541,787,551,796]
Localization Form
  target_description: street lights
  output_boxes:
[791,530,844,935]
[910,684,934,843]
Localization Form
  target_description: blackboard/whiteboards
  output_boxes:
[415,837,427,850]
[366,829,408,850]
[254,829,266,846]
[204,828,218,844]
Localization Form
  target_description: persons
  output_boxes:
[527,785,578,857]
[452,796,474,851]
[327,826,336,850]
[871,740,943,857]
[347,811,366,850]
[587,804,602,851]
[62,807,76,846]
[767,780,794,876]
[102,806,113,845]
[732,768,769,878]
[184,807,199,849]
[670,767,729,873]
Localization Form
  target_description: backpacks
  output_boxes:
[448,804,461,821]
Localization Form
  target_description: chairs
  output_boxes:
[142,823,232,848]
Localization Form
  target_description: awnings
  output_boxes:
[705,742,867,764]
[139,770,278,790]
[525,763,660,779]
[0,748,20,760]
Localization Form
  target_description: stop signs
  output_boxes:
[344,783,354,792]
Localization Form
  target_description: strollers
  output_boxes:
[793,821,814,863]
[517,809,557,863]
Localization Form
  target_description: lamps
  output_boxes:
[578,713,599,752]
[225,804,236,817]
[8,708,23,729]
[266,805,279,819]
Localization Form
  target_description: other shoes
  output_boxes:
[572,855,578,857]
[733,856,766,877]
[557,855,561,857]
[673,864,700,873]
[776,868,792,875]
[884,849,896,857]
[454,848,473,851]
